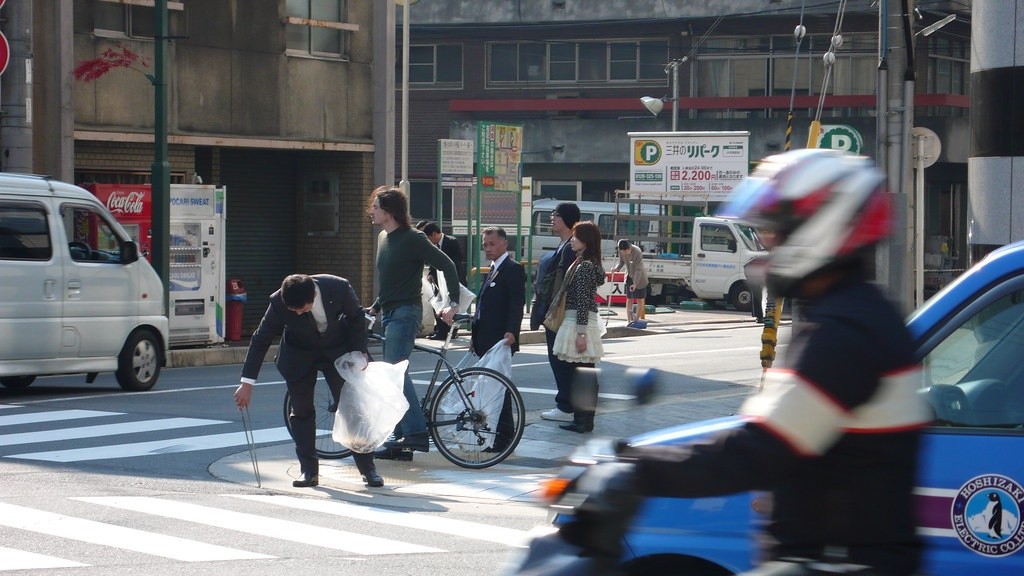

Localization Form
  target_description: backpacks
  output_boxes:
[535,244,571,298]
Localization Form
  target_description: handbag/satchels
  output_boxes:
[543,289,566,332]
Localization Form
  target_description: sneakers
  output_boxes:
[541,408,574,422]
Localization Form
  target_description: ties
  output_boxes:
[437,244,440,249]
[475,265,494,320]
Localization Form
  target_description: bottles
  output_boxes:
[170,233,195,263]
[97,231,139,259]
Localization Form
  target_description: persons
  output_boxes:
[552,147,930,576]
[415,220,467,341]
[529,201,605,433]
[233,273,385,487]
[361,188,460,453]
[471,226,526,453]
[611,239,649,327]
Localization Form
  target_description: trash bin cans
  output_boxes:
[226,279,246,340]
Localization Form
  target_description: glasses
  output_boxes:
[550,214,561,218]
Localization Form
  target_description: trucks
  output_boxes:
[598,216,770,312]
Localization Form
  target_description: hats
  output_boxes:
[553,203,581,230]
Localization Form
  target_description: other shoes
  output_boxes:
[365,470,384,487]
[293,473,318,487]
[426,332,446,339]
[375,448,413,461]
[384,433,429,452]
[560,420,595,434]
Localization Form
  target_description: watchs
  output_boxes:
[576,333,586,338]
[449,301,459,307]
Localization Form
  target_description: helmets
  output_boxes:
[715,147,894,284]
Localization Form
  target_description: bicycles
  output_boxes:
[284,309,525,469]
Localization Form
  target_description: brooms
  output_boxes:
[605,254,616,327]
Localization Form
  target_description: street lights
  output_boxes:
[640,95,678,132]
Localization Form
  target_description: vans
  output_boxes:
[0,171,171,391]
[523,196,666,274]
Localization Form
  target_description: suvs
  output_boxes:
[509,238,1024,576]
